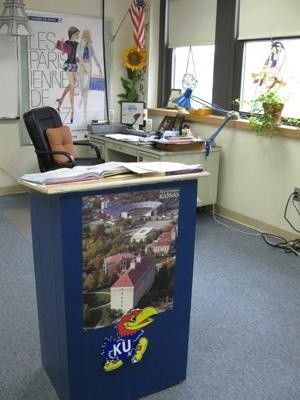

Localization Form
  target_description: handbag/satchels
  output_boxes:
[89,72,105,91]
[56,40,72,54]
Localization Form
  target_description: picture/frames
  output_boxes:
[166,89,181,110]
[158,116,175,133]
[172,115,185,134]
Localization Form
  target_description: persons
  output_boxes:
[75,30,103,119]
[55,26,80,124]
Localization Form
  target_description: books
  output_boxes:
[20,160,204,185]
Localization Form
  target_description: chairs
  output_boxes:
[23,106,103,173]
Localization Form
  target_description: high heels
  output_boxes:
[56,99,62,112]
[71,119,73,124]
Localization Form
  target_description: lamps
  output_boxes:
[0,0,36,37]
[174,89,235,154]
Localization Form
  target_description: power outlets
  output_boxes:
[293,186,300,202]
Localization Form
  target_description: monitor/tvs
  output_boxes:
[121,101,144,130]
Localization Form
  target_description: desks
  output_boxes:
[88,133,222,212]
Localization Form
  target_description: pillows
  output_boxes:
[45,127,77,163]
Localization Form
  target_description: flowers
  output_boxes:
[118,48,148,102]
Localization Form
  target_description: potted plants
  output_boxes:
[245,92,283,138]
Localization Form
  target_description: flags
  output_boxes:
[128,0,147,52]
